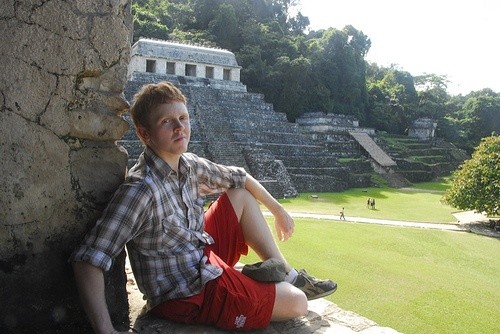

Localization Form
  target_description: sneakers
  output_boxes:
[293,269,338,301]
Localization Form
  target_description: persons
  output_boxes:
[367,198,376,210]
[340,207,345,221]
[67,82,337,334]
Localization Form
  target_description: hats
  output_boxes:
[241,256,288,282]
